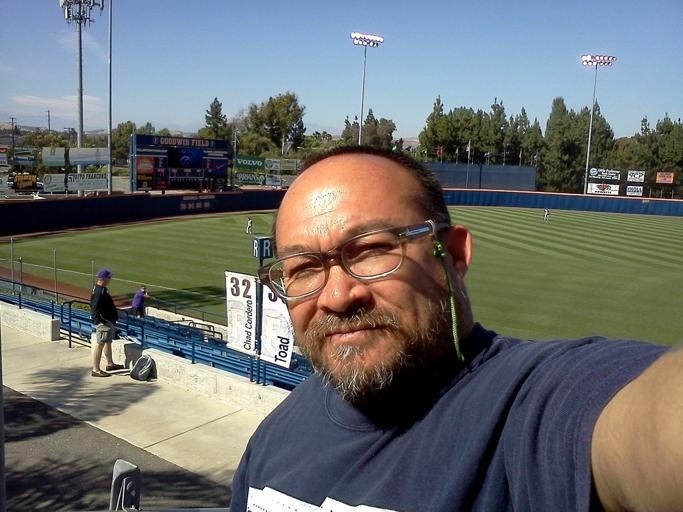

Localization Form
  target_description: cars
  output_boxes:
[36,181,43,189]
[7,172,15,188]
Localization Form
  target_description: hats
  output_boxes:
[95,267,116,280]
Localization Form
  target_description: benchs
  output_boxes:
[0,290,313,386]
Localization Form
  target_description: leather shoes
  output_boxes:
[105,363,123,371]
[91,369,110,377]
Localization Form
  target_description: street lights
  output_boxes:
[348,29,387,146]
[578,52,616,195]
[53,0,106,188]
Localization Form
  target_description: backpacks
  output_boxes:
[128,354,154,381]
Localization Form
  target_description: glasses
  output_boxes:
[255,222,449,305]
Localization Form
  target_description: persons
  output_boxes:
[543,208,551,224]
[230,144,683,512]
[132,286,153,318]
[245,218,253,235]
[90,269,124,377]
